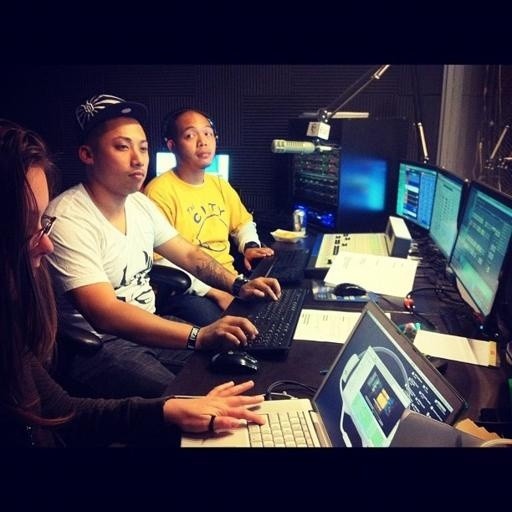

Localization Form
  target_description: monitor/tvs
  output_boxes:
[154,150,231,183]
[339,154,388,212]
[440,179,512,338]
[426,167,469,267]
[394,158,439,238]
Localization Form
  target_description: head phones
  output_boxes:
[159,107,218,142]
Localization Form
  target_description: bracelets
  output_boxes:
[232,277,249,298]
[187,325,201,351]
[244,241,260,253]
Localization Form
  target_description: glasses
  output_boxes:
[27,214,56,250]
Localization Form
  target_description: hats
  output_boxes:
[71,94,148,143]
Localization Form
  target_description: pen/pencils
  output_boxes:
[320,370,329,375]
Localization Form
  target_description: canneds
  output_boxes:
[293,208,306,232]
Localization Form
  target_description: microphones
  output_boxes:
[270,139,315,154]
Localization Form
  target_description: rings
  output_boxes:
[207,415,219,435]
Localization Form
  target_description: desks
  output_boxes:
[156,222,512,510]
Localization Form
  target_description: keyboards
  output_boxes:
[238,288,308,351]
[264,248,310,285]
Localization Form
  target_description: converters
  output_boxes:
[430,358,448,370]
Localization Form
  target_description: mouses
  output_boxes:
[334,283,366,296]
[208,350,259,374]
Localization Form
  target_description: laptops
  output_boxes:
[180,300,471,448]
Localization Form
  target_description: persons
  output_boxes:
[143,106,275,325]
[40,95,282,398]
[0,118,266,512]
